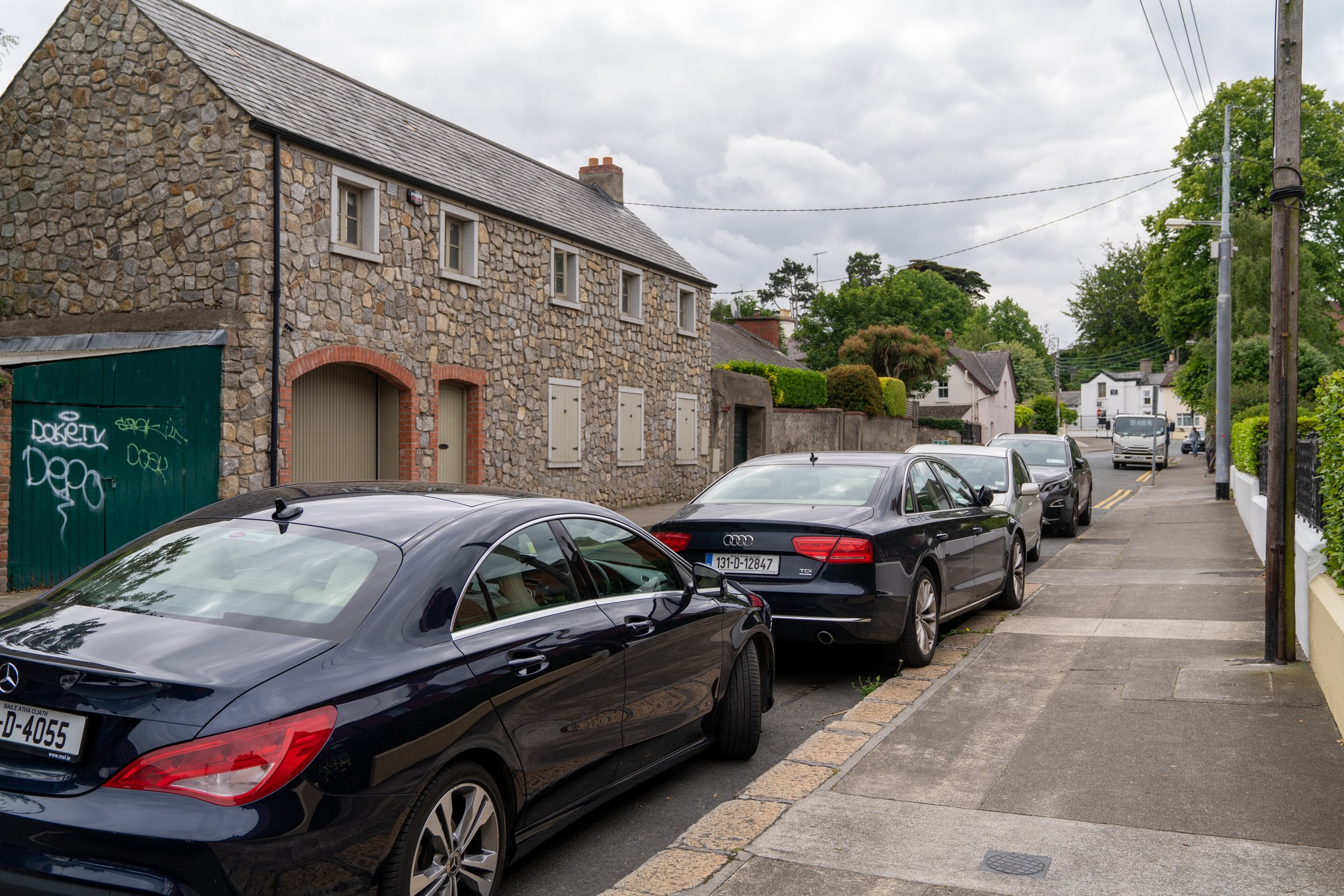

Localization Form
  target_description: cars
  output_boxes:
[904,443,1043,562]
[647,449,1027,670]
[1181,430,1206,454]
[0,478,776,896]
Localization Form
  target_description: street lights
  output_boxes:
[1055,339,1092,435]
[1184,340,1212,430]
[1164,214,1233,502]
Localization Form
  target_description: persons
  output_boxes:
[1204,425,1216,474]
[1189,427,1201,457]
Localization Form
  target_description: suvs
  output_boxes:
[970,432,1093,539]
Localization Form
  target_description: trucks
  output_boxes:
[1106,410,1175,471]
